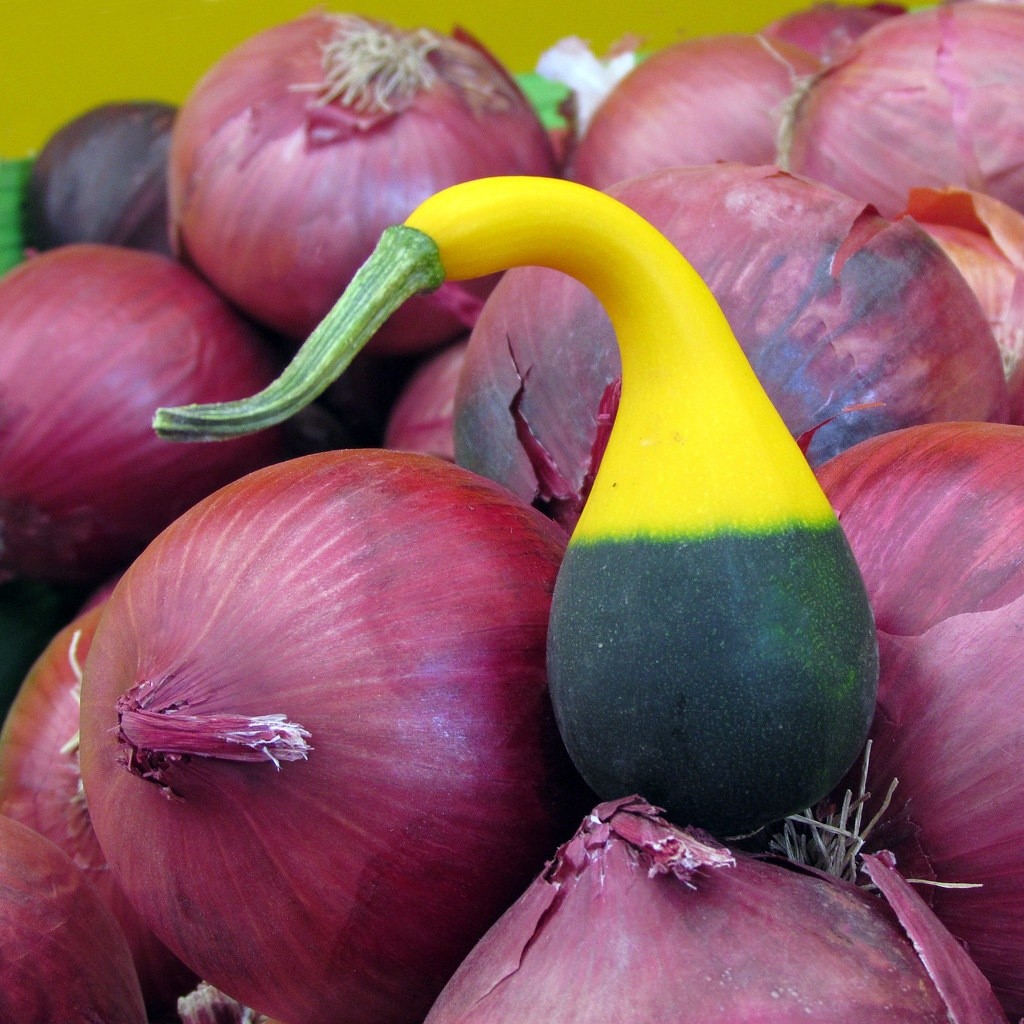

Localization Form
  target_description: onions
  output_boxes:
[1,1,1024,1024]
